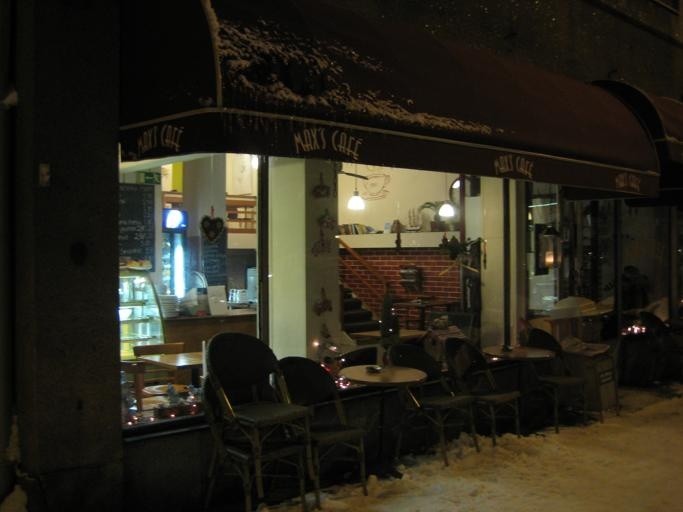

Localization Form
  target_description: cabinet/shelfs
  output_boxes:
[118,264,165,361]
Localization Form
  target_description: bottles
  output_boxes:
[229,289,249,305]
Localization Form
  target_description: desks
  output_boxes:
[338,365,428,479]
[560,340,621,415]
[481,343,556,436]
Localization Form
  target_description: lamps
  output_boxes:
[347,164,365,210]
[530,183,563,269]
[439,172,455,217]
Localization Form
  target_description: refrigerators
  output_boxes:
[161,208,189,302]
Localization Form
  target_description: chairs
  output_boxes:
[197,332,369,512]
[387,342,480,466]
[524,327,603,433]
[639,311,683,390]
[444,337,522,447]
[119,343,191,415]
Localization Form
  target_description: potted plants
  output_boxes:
[418,200,451,232]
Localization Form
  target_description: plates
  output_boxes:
[158,295,180,319]
